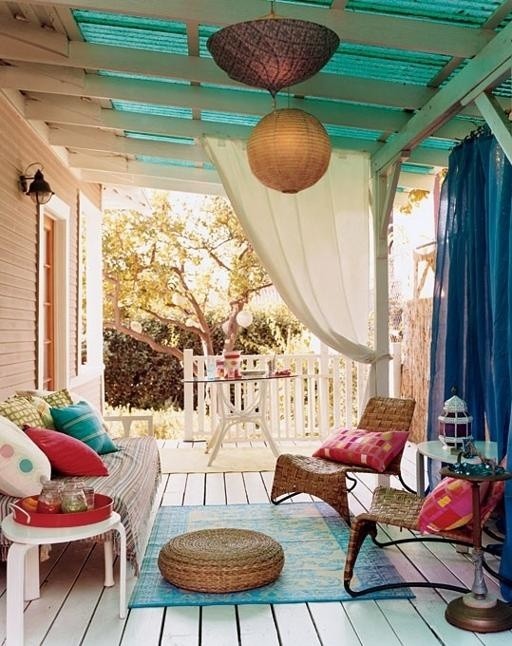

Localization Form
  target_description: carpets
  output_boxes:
[128,502,416,608]
[159,445,318,473]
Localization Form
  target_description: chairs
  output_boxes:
[271,396,417,528]
[344,454,512,606]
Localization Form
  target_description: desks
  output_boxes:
[181,376,299,467]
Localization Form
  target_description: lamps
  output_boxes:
[20,162,55,205]
[206,0,339,110]
[246,89,331,194]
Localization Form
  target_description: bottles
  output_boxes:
[207,363,215,380]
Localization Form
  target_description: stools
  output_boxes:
[0,511,126,646]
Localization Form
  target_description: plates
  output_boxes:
[240,369,268,375]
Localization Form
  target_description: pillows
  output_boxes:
[312,428,411,473]
[418,477,489,536]
[0,389,121,498]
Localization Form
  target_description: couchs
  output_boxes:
[1,415,162,588]
